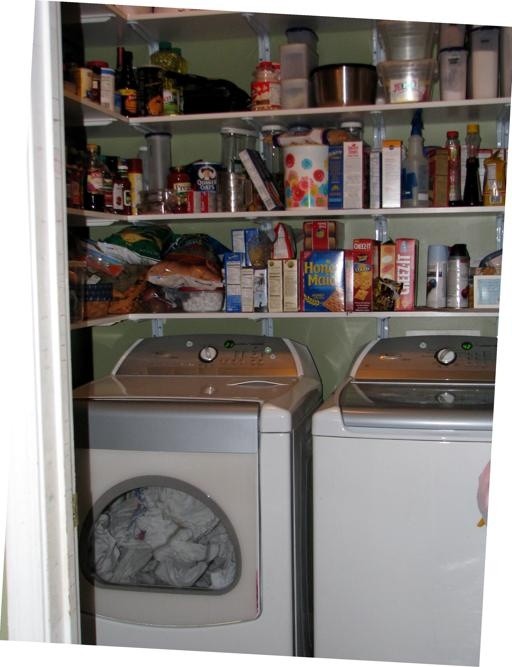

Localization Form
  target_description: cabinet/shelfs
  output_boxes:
[62,4,512,329]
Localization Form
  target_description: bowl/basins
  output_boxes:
[310,63,377,109]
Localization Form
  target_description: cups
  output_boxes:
[444,131,462,206]
[145,133,172,190]
[218,172,253,212]
[168,165,193,213]
[261,124,286,175]
[68,261,84,324]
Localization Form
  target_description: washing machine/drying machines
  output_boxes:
[312,336,490,666]
[73,335,332,658]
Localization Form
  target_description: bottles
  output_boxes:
[113,166,132,215]
[342,122,363,140]
[86,145,105,211]
[494,105,509,149]
[447,244,471,309]
[463,124,484,204]
[149,41,180,116]
[426,244,450,309]
[233,116,259,170]
[116,47,125,78]
[139,146,150,193]
[375,217,388,244]
[121,52,138,117]
